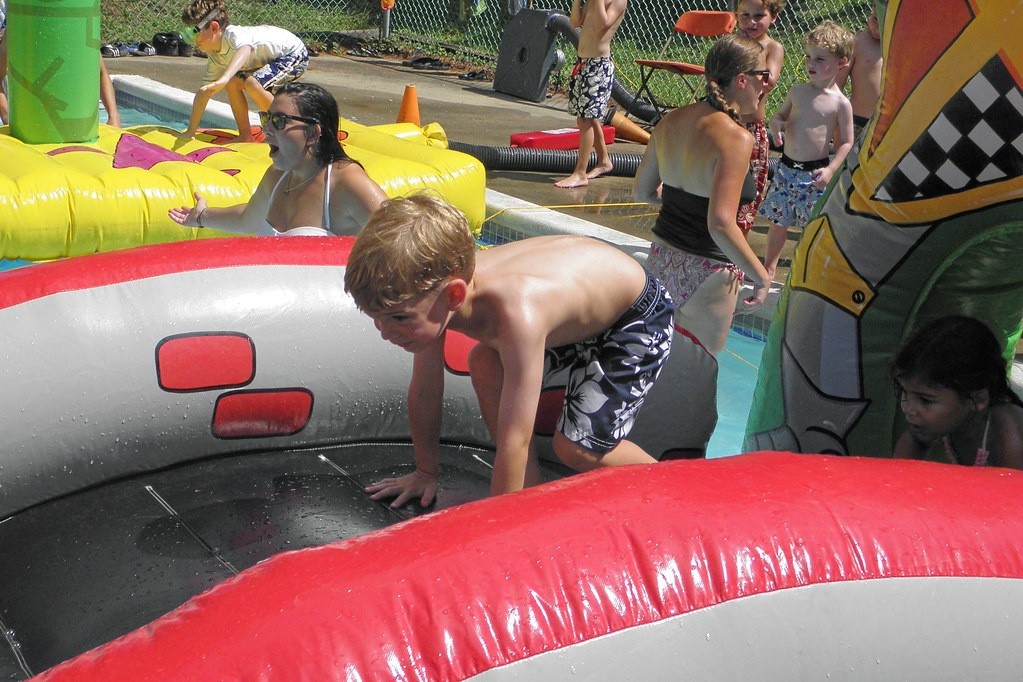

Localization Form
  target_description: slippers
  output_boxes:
[403,58,451,69]
[194,50,208,58]
[458,70,493,82]
[101,40,157,58]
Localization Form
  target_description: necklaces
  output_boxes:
[283,166,322,194]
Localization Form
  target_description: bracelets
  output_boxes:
[196,207,207,228]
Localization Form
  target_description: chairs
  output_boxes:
[619,12,736,134]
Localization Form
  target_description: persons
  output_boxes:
[891,315,1022,472]
[554,0,626,187]
[167,82,390,235]
[344,194,676,508]
[833,0,902,142]
[178,0,309,142]
[630,34,772,357]
[0,16,121,129]
[764,20,854,281]
[733,0,788,240]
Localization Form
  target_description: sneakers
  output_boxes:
[153,31,194,57]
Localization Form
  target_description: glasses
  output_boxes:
[179,8,221,47]
[747,69,769,83]
[259,111,323,136]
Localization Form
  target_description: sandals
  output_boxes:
[347,42,382,57]
[306,45,320,56]
